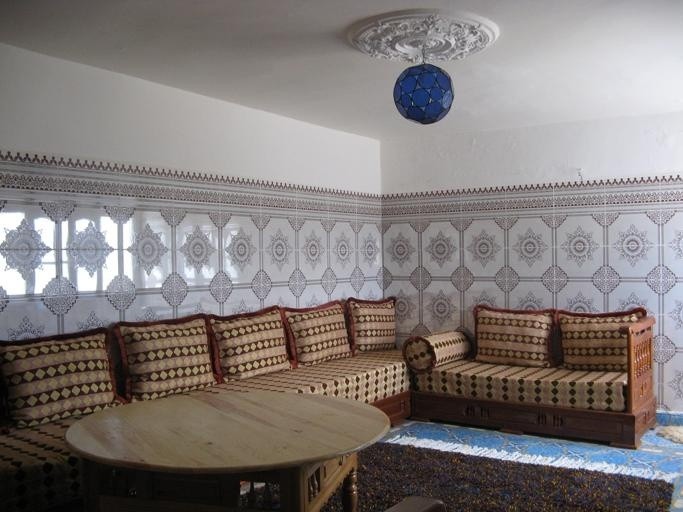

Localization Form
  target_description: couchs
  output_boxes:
[1,348,410,512]
[401,318,657,450]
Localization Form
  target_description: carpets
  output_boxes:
[63,442,673,512]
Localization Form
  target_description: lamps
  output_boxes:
[345,7,499,126]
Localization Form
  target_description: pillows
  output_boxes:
[404,329,469,368]
[348,297,397,351]
[282,299,352,367]
[1,326,129,426]
[470,303,555,367]
[207,304,294,382]
[113,312,218,400]
[558,308,647,371]
